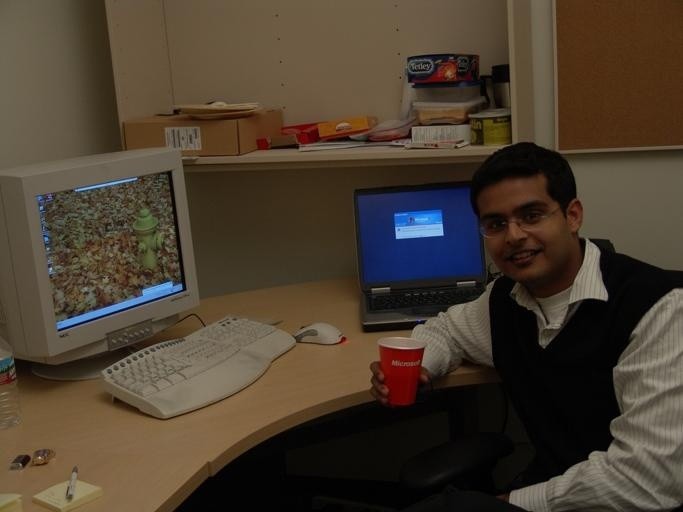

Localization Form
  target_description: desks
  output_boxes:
[0,277,492,512]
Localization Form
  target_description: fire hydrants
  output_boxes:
[133,208,165,270]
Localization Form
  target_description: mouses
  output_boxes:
[294,321,344,345]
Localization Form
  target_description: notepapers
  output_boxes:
[33,478,102,512]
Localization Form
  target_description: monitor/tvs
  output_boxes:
[0,148,201,381]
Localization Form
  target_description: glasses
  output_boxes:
[478,201,562,238]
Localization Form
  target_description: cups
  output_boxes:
[491,64,511,108]
[377,337,428,406]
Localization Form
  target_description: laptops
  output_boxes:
[353,179,489,331]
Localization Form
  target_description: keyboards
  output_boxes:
[100,314,296,420]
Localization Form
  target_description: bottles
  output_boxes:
[0,336,21,430]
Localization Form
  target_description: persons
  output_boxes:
[368,141,683,511]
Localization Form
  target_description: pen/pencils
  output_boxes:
[66,465,78,501]
